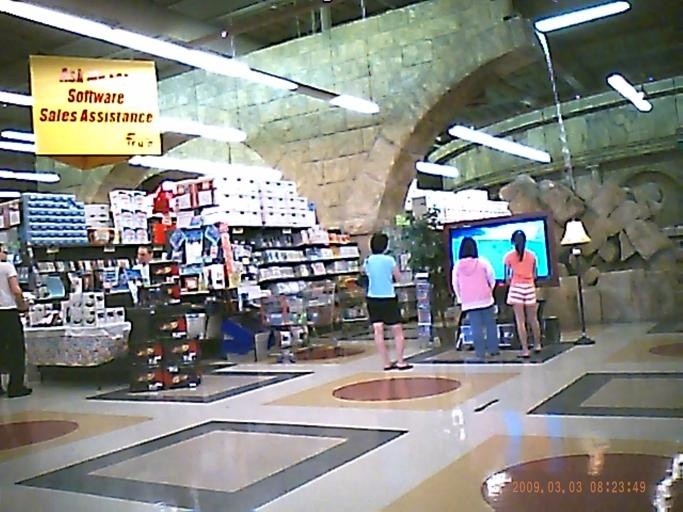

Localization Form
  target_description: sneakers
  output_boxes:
[8,389,32,398]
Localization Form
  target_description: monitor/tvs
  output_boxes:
[448,213,551,285]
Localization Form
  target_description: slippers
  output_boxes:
[385,363,413,370]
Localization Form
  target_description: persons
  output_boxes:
[360,233,413,369]
[451,237,501,359]
[503,230,542,357]
[0,243,32,398]
[132,246,154,287]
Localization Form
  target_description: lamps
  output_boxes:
[561,219,596,345]
[0,1,380,115]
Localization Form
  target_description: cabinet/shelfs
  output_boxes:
[455,300,556,350]
